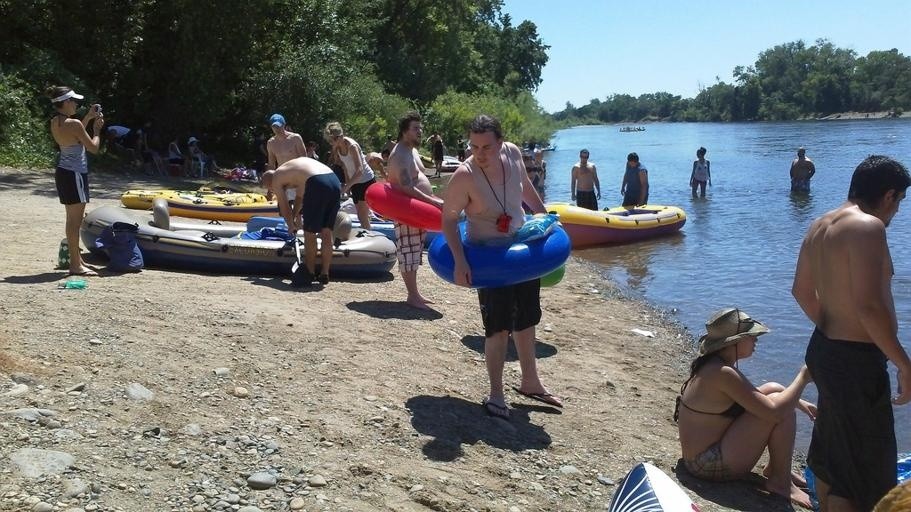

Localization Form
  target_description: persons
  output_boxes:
[108,121,222,177]
[570,149,602,210]
[669,308,819,507]
[787,146,816,193]
[49,85,105,276]
[254,112,465,311]
[789,154,910,511]
[441,115,565,420]
[622,151,650,206]
[517,142,547,210]
[689,148,712,198]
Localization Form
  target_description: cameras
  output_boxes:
[93,103,102,115]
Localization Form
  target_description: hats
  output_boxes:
[50,86,85,103]
[270,114,286,128]
[698,308,769,358]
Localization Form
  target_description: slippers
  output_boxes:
[513,385,563,407]
[483,399,510,420]
[70,265,98,276]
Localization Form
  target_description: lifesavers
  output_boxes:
[428,214,572,288]
[364,182,443,233]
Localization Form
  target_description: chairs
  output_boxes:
[190,150,209,177]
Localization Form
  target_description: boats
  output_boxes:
[418,154,435,167]
[120,186,295,221]
[181,162,269,195]
[235,213,454,248]
[537,200,687,248]
[440,155,462,172]
[619,127,646,132]
[79,206,393,280]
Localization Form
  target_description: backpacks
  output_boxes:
[94,222,145,273]
